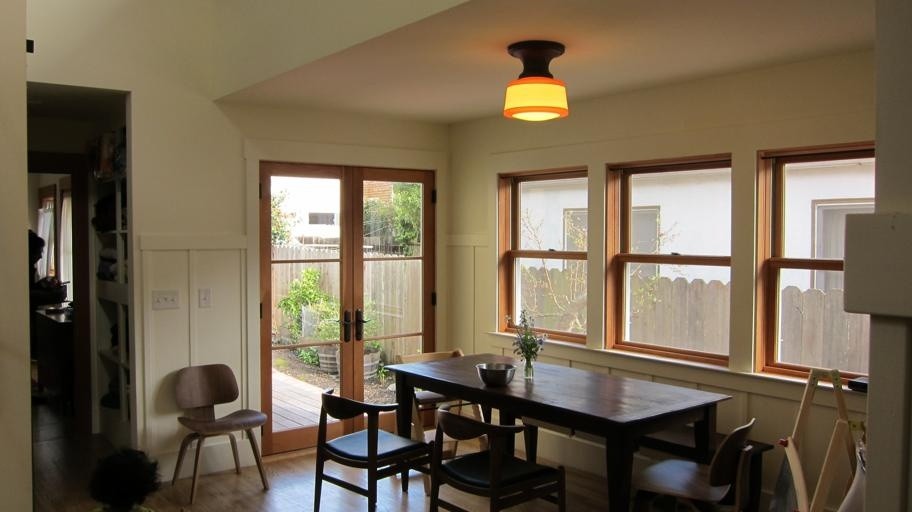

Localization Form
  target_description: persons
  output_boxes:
[30,228,69,306]
[86,445,161,511]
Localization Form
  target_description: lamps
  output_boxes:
[502,39,570,124]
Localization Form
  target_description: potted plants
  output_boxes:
[313,317,339,373]
[332,300,381,381]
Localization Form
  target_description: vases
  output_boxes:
[521,357,534,379]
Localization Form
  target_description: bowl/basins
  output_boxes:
[474,362,519,388]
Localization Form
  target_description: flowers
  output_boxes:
[506,307,548,360]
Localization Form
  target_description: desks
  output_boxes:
[35,297,84,405]
[384,350,733,512]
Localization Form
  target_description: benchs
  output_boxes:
[520,410,776,488]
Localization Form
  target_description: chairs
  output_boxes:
[624,408,758,511]
[170,361,270,505]
[429,401,569,511]
[312,385,440,512]
[394,347,485,497]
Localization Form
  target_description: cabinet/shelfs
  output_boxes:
[85,166,135,446]
